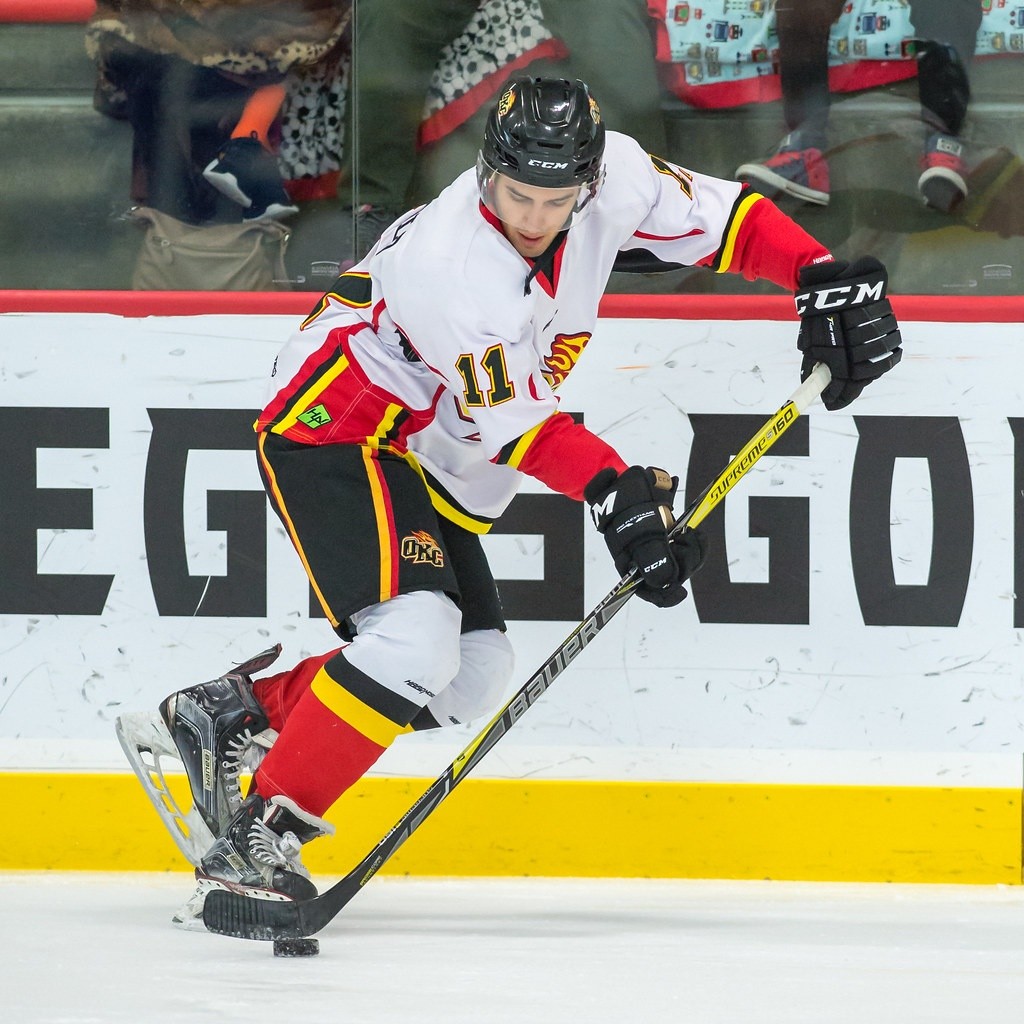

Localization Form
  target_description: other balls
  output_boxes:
[273,938,320,958]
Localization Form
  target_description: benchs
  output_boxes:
[0,0,1024,294]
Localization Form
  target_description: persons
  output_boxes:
[340,0,668,274]
[123,74,904,935]
[735,0,983,216]
[83,0,353,225]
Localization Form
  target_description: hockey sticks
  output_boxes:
[201,361,834,942]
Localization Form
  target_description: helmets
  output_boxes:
[479,75,605,198]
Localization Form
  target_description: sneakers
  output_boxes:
[242,187,300,224]
[158,642,281,840]
[734,130,831,208]
[917,133,968,214]
[203,131,274,208]
[193,793,334,903]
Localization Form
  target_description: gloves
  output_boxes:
[794,257,905,412]
[591,466,706,608]
